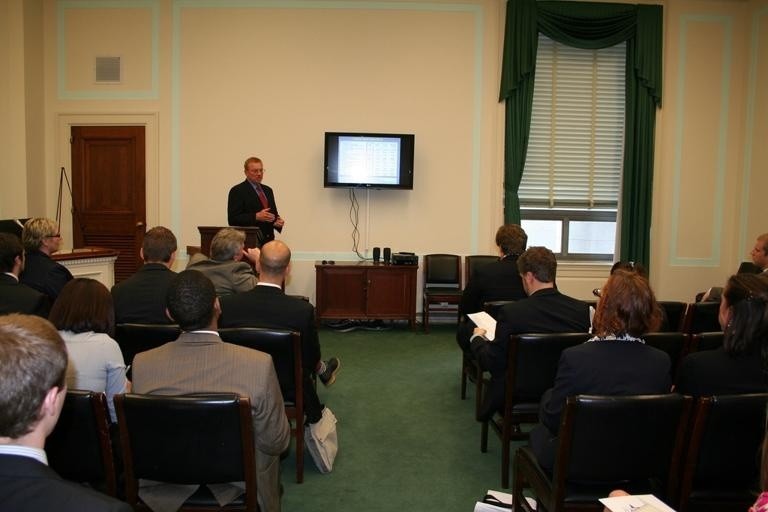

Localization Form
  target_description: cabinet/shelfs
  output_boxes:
[314,260,419,332]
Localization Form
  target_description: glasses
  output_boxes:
[247,169,265,173]
[592,287,605,301]
[46,233,60,237]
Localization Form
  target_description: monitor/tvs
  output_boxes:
[321,132,414,191]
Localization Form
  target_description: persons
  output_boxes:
[131,272,290,504]
[1,234,41,315]
[43,279,132,425]
[23,220,72,291]
[2,317,130,511]
[700,236,767,302]
[186,228,262,300]
[589,260,646,332]
[111,228,181,322]
[520,270,676,491]
[674,274,767,392]
[227,157,285,248]
[468,249,590,419]
[456,224,537,360]
[602,434,767,512]
[218,240,338,426]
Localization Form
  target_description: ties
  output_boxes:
[256,185,268,209]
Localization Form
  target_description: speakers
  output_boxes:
[373,247,381,262]
[383,247,391,265]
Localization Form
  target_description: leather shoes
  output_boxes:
[496,419,521,433]
[319,357,341,387]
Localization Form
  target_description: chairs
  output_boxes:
[43,388,119,512]
[502,392,695,512]
[479,332,594,491]
[112,391,261,512]
[459,300,517,423]
[684,300,722,332]
[216,325,304,485]
[580,300,597,310]
[422,253,463,335]
[657,300,689,331]
[640,331,692,387]
[464,254,501,290]
[693,330,724,391]
[691,392,768,511]
[287,295,310,303]
[115,322,182,382]
